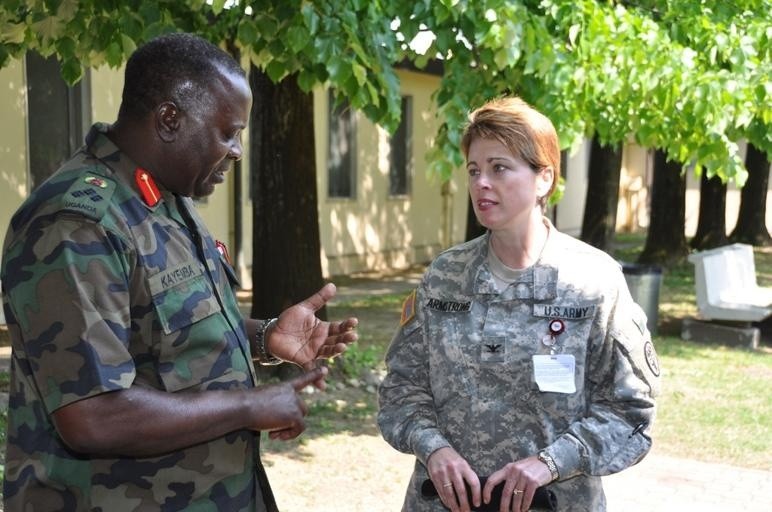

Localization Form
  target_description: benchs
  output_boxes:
[685,240,772,329]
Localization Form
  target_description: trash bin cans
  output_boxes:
[623,264,662,331]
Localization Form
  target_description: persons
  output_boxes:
[378,93,659,512]
[0,33,359,512]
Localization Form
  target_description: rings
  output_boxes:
[513,488,525,495]
[443,483,453,488]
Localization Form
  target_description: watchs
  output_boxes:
[255,318,284,366]
[538,450,559,480]
